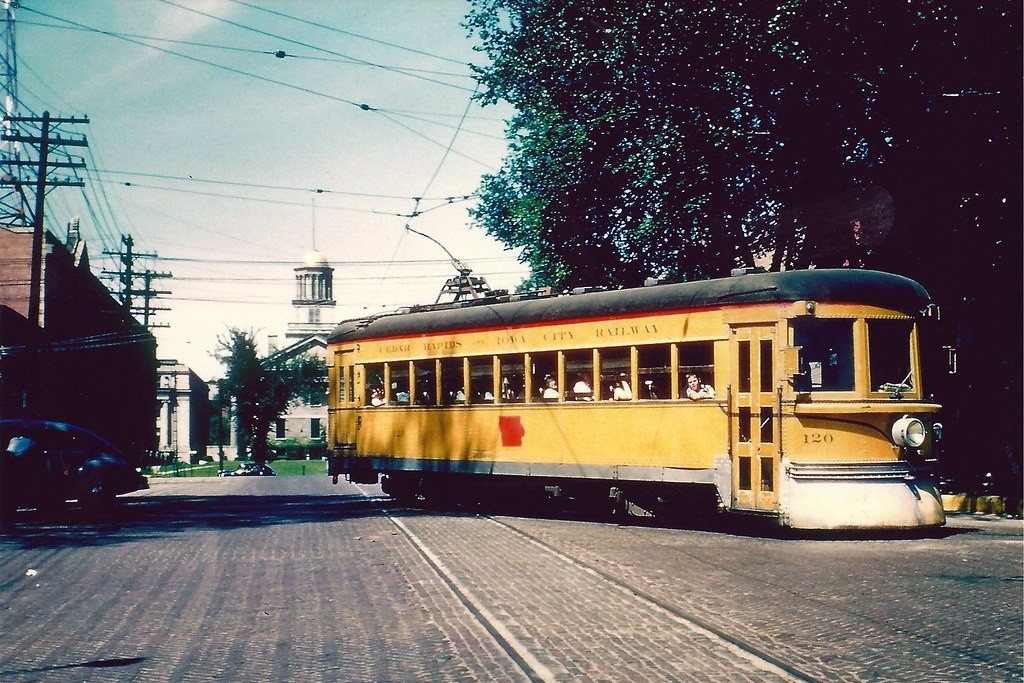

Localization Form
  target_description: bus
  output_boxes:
[326,267,958,532]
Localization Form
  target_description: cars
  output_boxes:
[218,463,275,477]
[0,420,148,525]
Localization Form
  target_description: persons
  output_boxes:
[358,368,714,404]
